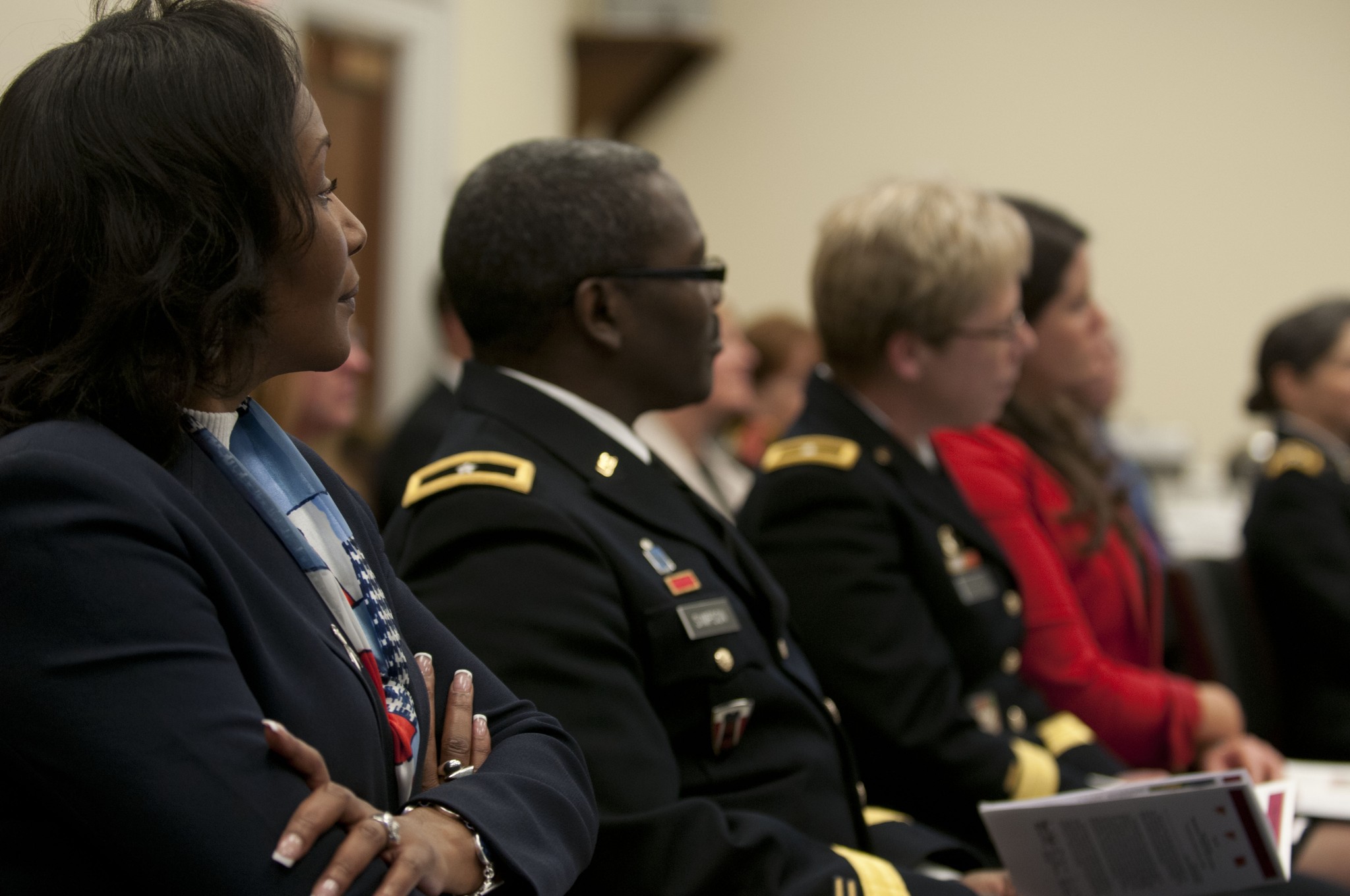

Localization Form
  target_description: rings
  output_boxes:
[438,759,465,776]
[369,811,401,851]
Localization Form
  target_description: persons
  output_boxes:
[368,267,823,519]
[1226,301,1349,761]
[243,314,391,505]
[0,0,599,896]
[739,184,1174,876]
[373,136,1017,896]
[932,196,1350,888]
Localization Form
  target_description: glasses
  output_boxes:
[575,257,726,288]
[954,309,1025,343]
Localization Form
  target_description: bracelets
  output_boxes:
[401,804,499,896]
